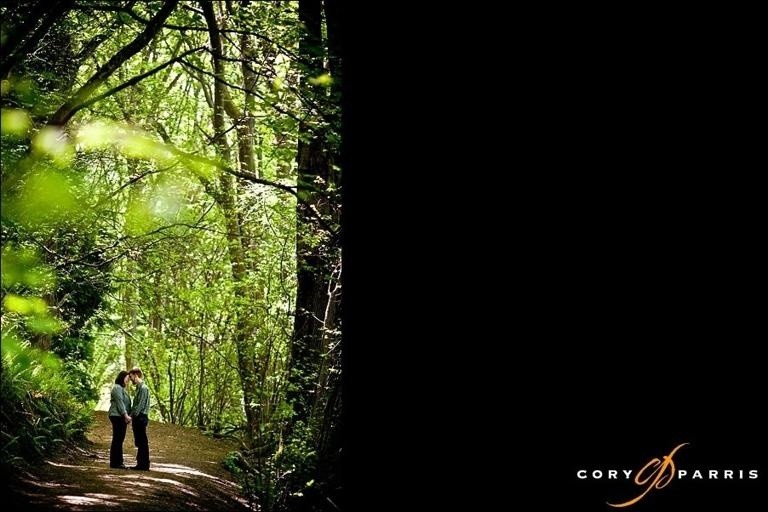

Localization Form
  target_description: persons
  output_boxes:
[108,369,132,471]
[123,368,150,470]
